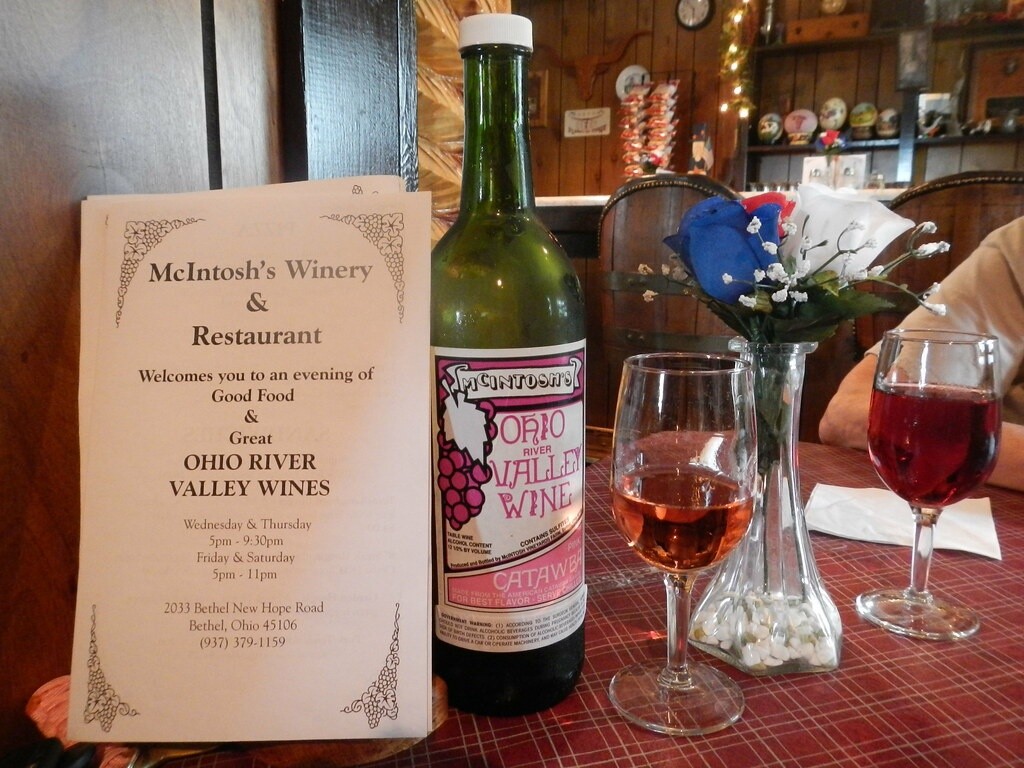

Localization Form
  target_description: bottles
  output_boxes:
[429,11,584,715]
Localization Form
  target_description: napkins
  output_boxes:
[802,481,1002,563]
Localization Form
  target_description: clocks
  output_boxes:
[674,0,714,30]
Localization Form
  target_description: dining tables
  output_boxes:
[22,424,1022,767]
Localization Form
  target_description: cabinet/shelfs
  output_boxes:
[636,69,697,183]
[728,2,1024,192]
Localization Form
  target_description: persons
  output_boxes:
[818,213,1024,491]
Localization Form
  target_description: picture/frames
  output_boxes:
[524,68,549,129]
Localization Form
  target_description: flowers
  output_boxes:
[629,184,954,480]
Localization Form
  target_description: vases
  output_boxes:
[679,332,849,679]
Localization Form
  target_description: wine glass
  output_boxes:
[605,351,758,734]
[854,326,1000,641]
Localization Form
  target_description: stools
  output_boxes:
[597,176,765,420]
[856,171,1024,365]
